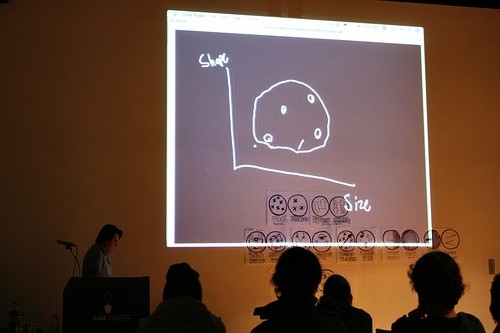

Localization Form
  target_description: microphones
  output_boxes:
[56,240,77,247]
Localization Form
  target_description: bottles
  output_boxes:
[16,314,27,333]
[8,301,20,333]
[35,320,43,333]
[30,316,36,333]
[48,314,60,333]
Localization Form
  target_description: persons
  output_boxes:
[391,251,486,333]
[489,274,500,333]
[249,247,348,333]
[137,262,226,333]
[317,274,372,333]
[82,224,123,277]
